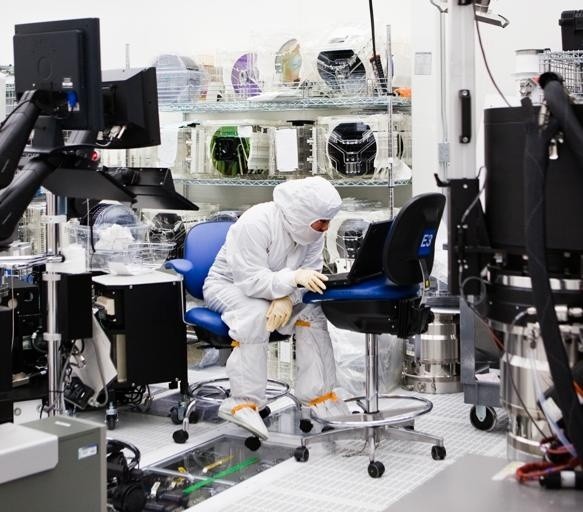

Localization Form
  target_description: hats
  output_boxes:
[273,176,342,245]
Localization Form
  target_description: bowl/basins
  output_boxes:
[94,241,177,275]
[62,222,150,266]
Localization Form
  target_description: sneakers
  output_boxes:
[217,398,268,440]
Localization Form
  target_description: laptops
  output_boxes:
[297,219,393,289]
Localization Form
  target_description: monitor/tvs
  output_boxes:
[14,18,104,155]
[96,67,161,149]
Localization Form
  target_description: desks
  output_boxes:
[56,263,199,429]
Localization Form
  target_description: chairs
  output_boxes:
[163,221,311,451]
[294,192,446,477]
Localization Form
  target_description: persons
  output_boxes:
[203,176,352,441]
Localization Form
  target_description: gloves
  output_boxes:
[266,296,293,332]
[295,270,328,294]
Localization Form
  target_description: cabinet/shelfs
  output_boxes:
[1,25,413,343]
